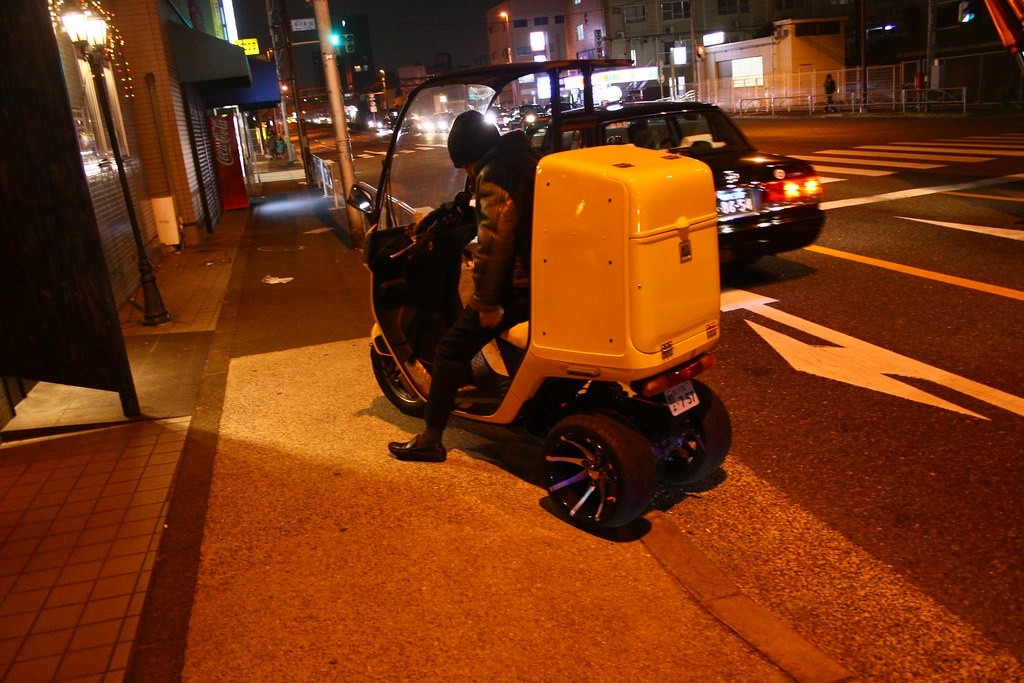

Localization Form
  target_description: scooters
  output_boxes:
[343,58,733,530]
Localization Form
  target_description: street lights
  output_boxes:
[499,12,518,106]
[61,0,171,327]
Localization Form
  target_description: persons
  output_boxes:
[266,126,278,160]
[824,74,837,113]
[389,110,545,462]
[627,123,648,145]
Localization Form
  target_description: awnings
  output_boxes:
[165,17,281,112]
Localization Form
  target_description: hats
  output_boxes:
[448,110,500,168]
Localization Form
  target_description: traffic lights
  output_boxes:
[331,34,339,45]
[346,34,354,51]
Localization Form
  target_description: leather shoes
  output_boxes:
[388,434,446,461]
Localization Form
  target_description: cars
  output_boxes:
[347,102,585,133]
[523,102,825,261]
